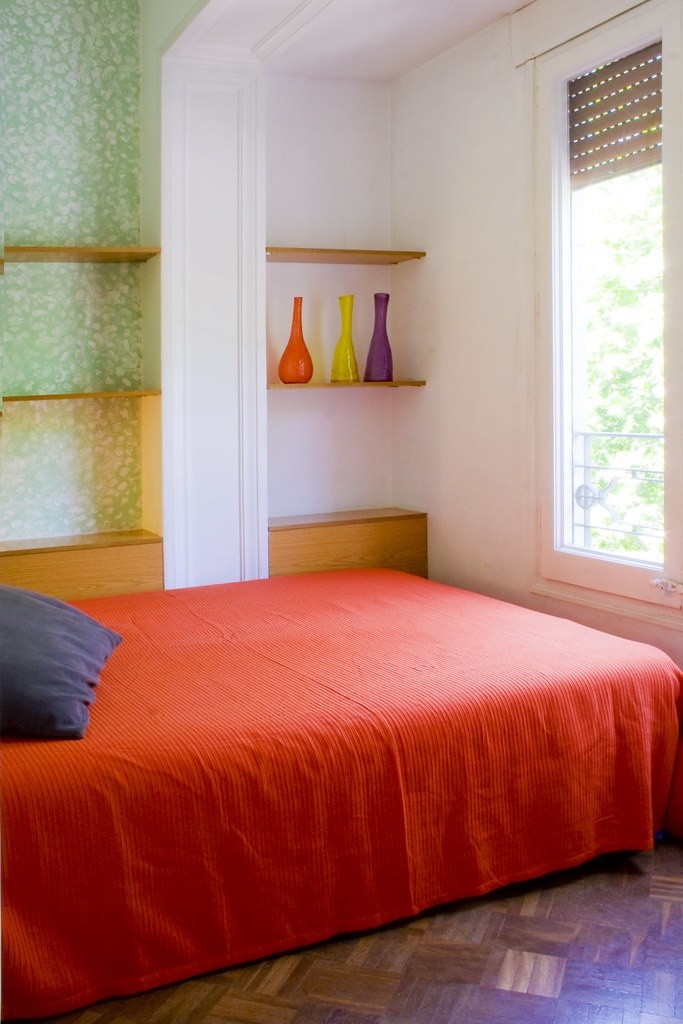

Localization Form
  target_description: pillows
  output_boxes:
[1,582,125,741]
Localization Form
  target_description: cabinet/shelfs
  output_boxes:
[2,245,159,401]
[264,245,426,388]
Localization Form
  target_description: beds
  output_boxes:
[0,565,683,1020]
